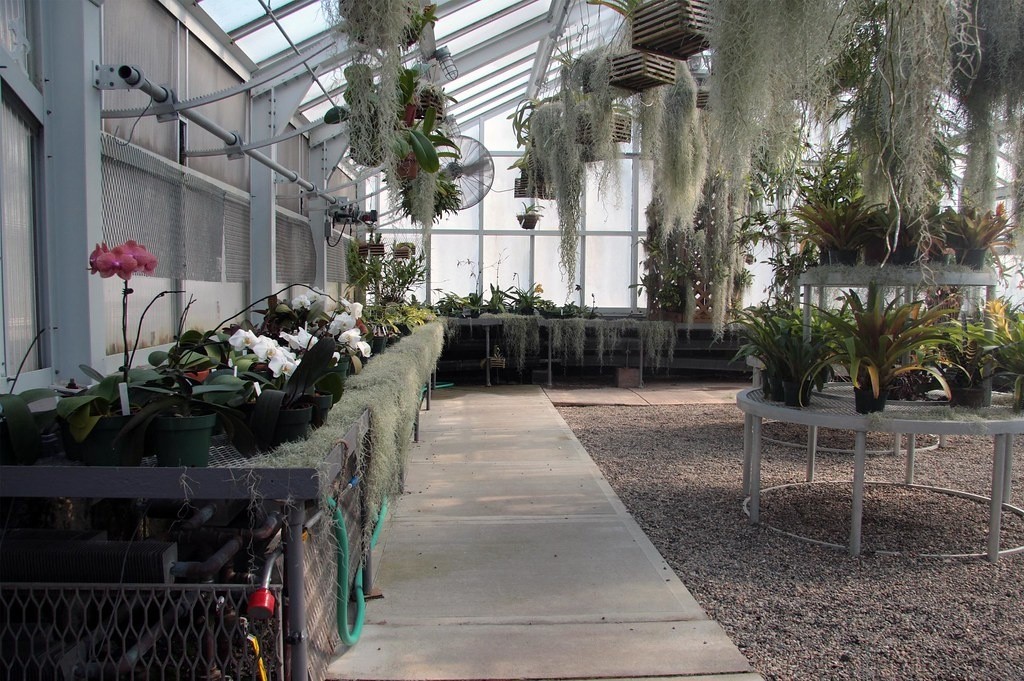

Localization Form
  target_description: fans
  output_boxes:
[432,135,494,210]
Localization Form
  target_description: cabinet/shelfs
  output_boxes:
[736,266,1024,563]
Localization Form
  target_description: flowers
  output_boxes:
[54,240,373,450]
[505,283,542,312]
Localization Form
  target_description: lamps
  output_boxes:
[444,115,461,138]
[434,46,459,82]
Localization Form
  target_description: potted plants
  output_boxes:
[479,345,506,368]
[506,0,717,229]
[390,240,416,259]
[319,0,458,223]
[369,233,385,256]
[432,273,603,317]
[349,239,368,257]
[721,194,1024,413]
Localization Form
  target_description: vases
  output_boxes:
[315,357,349,404]
[372,336,389,355]
[241,403,312,455]
[149,408,217,467]
[75,413,141,466]
[287,393,333,431]
[615,368,639,388]
